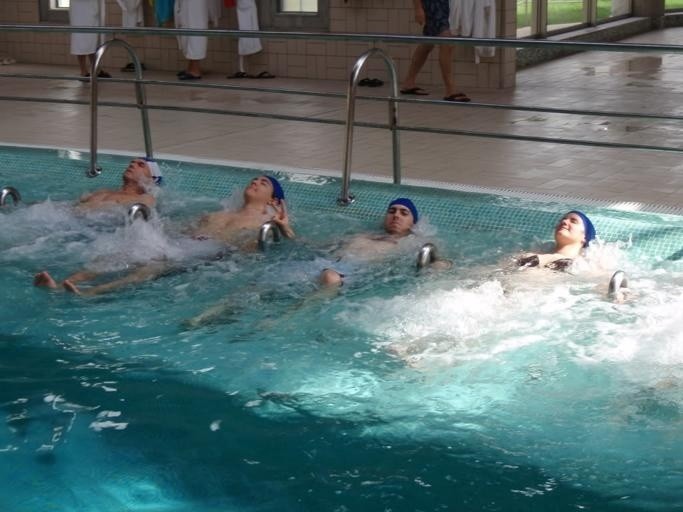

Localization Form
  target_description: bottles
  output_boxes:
[567,211,596,248]
[265,176,284,203]
[390,198,418,224]
[140,156,161,186]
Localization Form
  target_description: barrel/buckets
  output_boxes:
[400,87,428,95]
[121,63,146,71]
[357,79,383,86]
[443,93,469,101]
[82,70,111,77]
[177,71,201,80]
[227,71,277,79]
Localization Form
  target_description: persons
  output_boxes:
[399,0,472,102]
[32,174,296,297]
[188,198,454,334]
[69,0,112,81]
[71,158,162,216]
[384,211,624,369]
[175,0,208,80]
[117,0,148,71]
[227,2,276,79]
